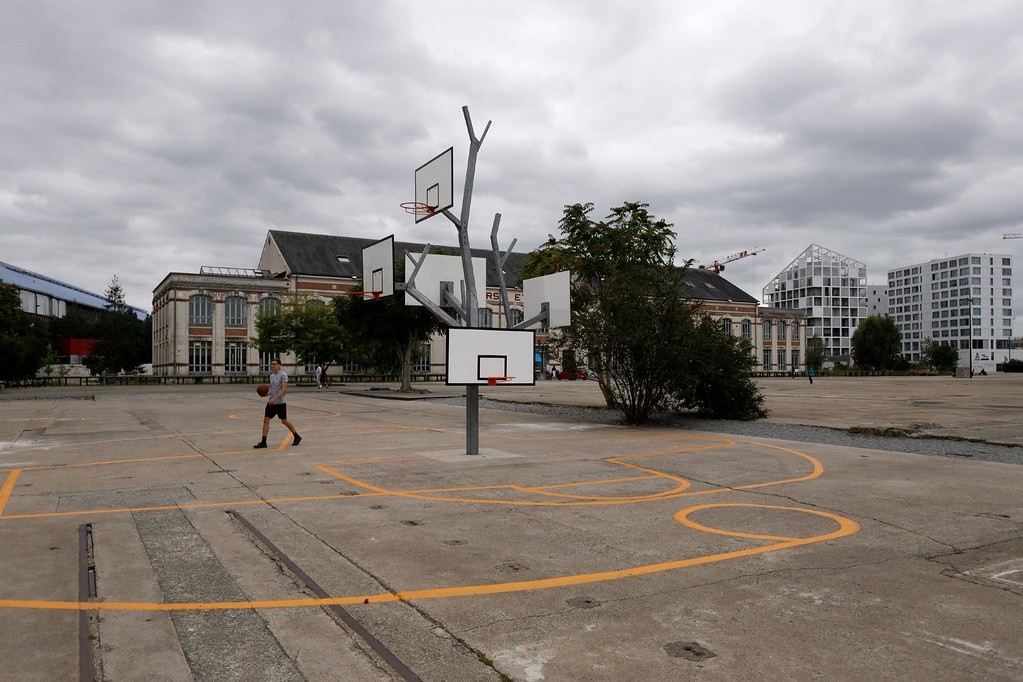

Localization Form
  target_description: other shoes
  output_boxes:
[326,387,329,388]
[317,387,320,389]
[292,436,302,446]
[253,443,267,448]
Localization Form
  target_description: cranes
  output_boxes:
[1002,233,1023,239]
[708,247,765,275]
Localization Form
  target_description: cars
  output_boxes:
[556,366,588,381]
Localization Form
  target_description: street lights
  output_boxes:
[966,298,973,378]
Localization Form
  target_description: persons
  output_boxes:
[808,365,814,384]
[552,365,556,379]
[315,363,329,389]
[253,359,302,448]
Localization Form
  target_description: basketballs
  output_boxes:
[256,384,269,396]
